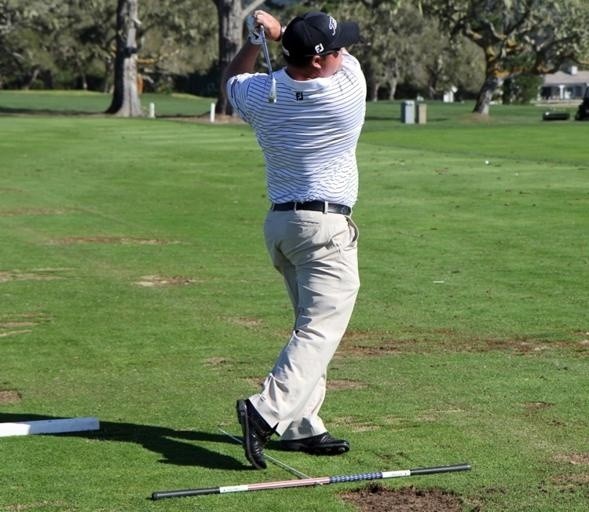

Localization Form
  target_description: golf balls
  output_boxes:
[485,160,489,163]
[545,112,549,115]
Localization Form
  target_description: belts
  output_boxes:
[274,201,351,216]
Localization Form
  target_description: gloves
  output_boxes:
[246,15,263,45]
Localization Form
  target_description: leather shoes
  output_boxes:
[236,399,276,470]
[280,431,350,456]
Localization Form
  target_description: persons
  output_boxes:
[220,10,368,470]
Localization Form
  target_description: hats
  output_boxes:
[282,11,360,64]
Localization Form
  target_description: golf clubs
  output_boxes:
[259,24,277,104]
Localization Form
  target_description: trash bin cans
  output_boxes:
[401,100,427,124]
